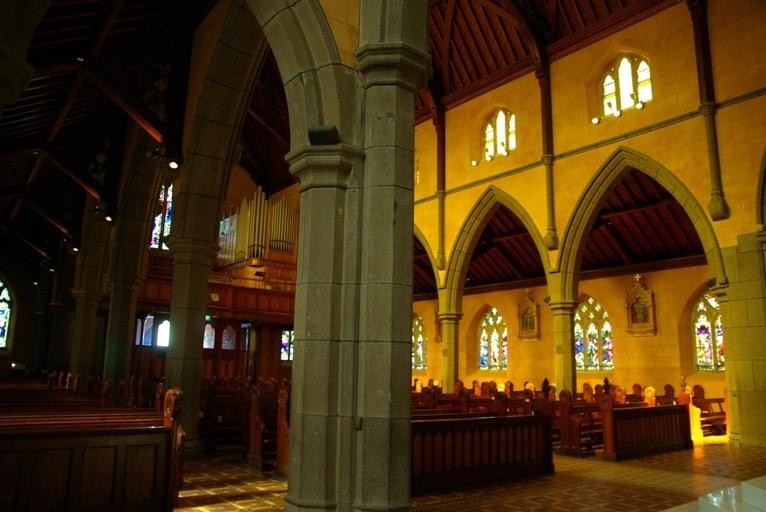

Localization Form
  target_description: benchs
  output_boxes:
[0,369,726,511]
